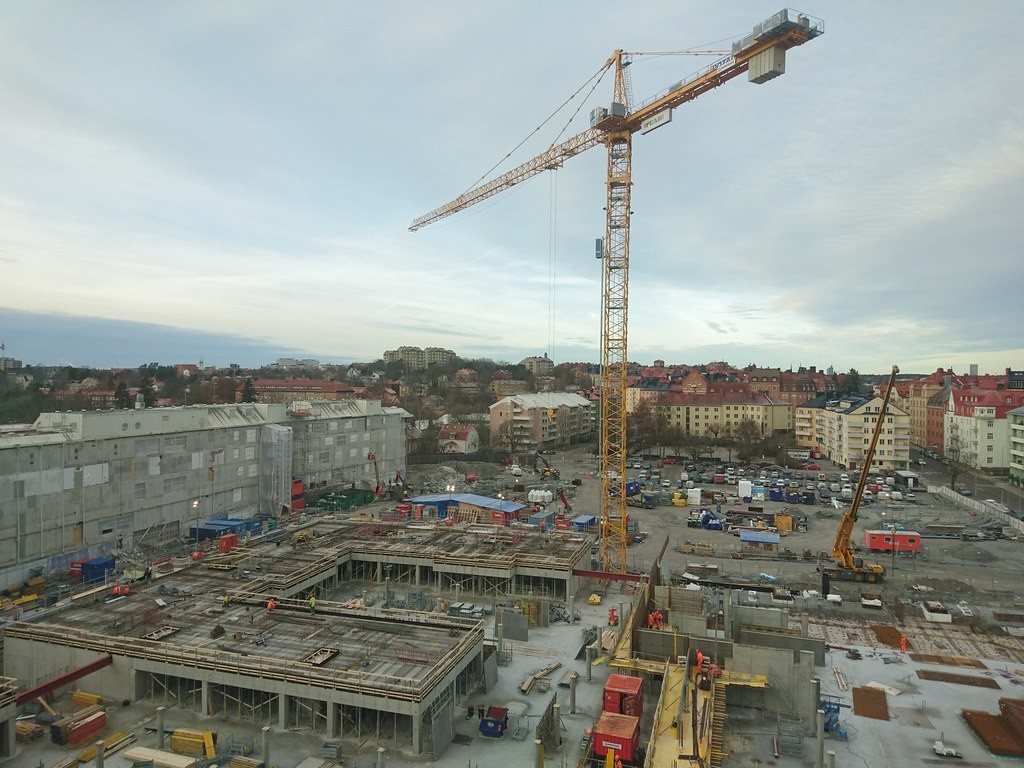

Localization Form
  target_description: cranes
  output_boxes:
[408,8,824,589]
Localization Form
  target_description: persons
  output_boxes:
[648,613,655,629]
[222,593,232,607]
[112,584,129,598]
[696,648,703,674]
[193,550,202,561]
[309,594,316,616]
[404,488,407,498]
[541,519,545,533]
[655,610,663,630]
[268,599,276,612]
[900,634,906,654]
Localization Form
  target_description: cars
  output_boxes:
[918,459,926,465]
[920,448,951,465]
[608,455,917,509]
[958,486,972,496]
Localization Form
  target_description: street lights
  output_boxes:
[920,446,938,484]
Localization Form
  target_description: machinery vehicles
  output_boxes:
[533,451,561,482]
[367,452,408,501]
[824,364,900,583]
[554,488,572,530]
